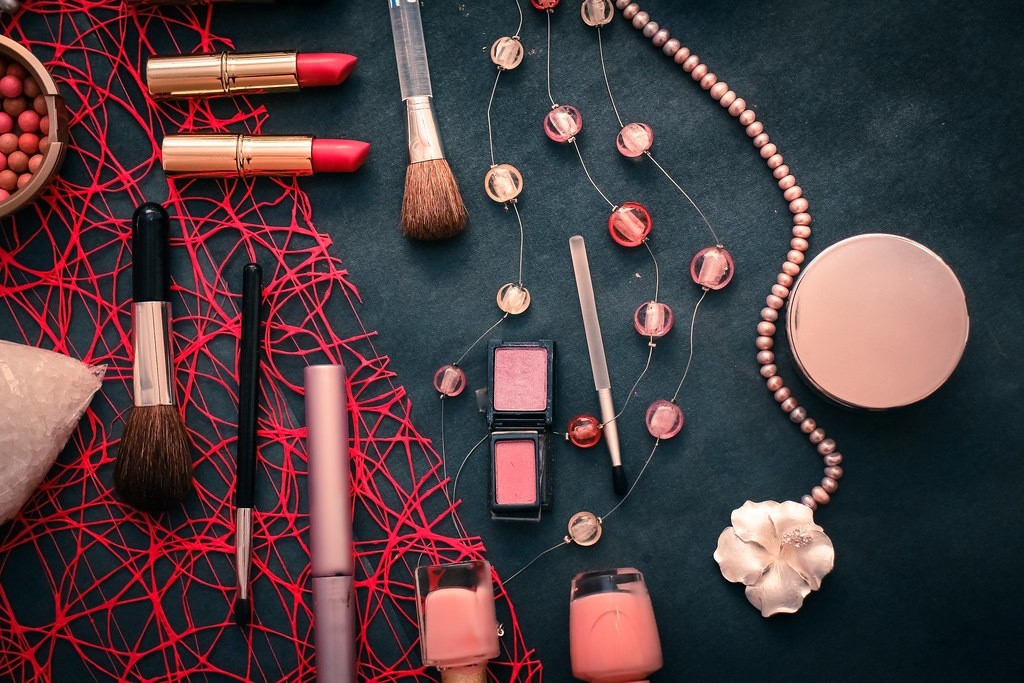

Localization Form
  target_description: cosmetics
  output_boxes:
[785,232,970,412]
[415,560,499,682]
[570,567,663,682]
[485,338,555,523]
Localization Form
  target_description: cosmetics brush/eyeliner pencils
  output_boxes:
[387,1,469,240]
[569,235,630,499]
[114,202,193,498]
[233,264,261,627]
[302,364,359,682]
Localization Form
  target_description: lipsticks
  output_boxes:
[161,134,370,180]
[146,50,358,101]
